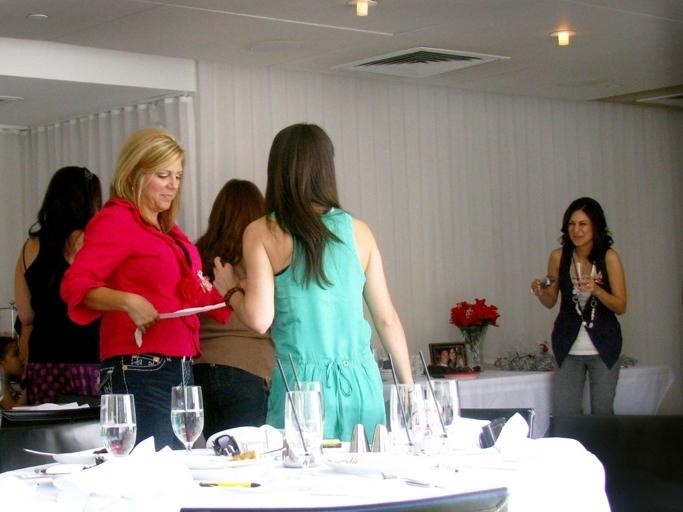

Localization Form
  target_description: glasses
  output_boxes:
[213,434,239,456]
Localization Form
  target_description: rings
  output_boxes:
[586,282,591,288]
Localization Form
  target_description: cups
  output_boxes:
[576,262,595,291]
[410,354,423,378]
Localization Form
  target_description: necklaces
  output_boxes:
[569,247,600,328]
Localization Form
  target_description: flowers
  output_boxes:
[448,296,499,347]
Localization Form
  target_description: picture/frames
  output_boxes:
[429,342,465,371]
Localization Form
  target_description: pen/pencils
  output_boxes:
[199,481,262,488]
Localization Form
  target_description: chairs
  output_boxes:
[549,417,683,512]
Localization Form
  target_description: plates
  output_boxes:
[52,452,96,461]
[381,368,418,381]
[46,464,82,475]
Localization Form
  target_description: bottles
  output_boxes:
[369,424,391,453]
[349,424,371,452]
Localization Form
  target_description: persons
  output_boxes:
[528,197,626,419]
[58,124,252,454]
[1,164,105,415]
[182,167,276,441]
[211,123,414,455]
[437,348,463,369]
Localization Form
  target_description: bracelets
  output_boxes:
[223,287,245,311]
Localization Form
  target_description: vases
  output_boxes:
[462,338,484,371]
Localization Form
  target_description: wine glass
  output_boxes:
[99,393,137,458]
[425,380,459,437]
[282,391,321,468]
[296,383,325,448]
[170,386,205,456]
[389,384,425,444]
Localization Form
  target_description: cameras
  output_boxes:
[543,275,556,289]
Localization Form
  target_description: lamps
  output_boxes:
[356,0,368,16]
[557,31,569,46]
